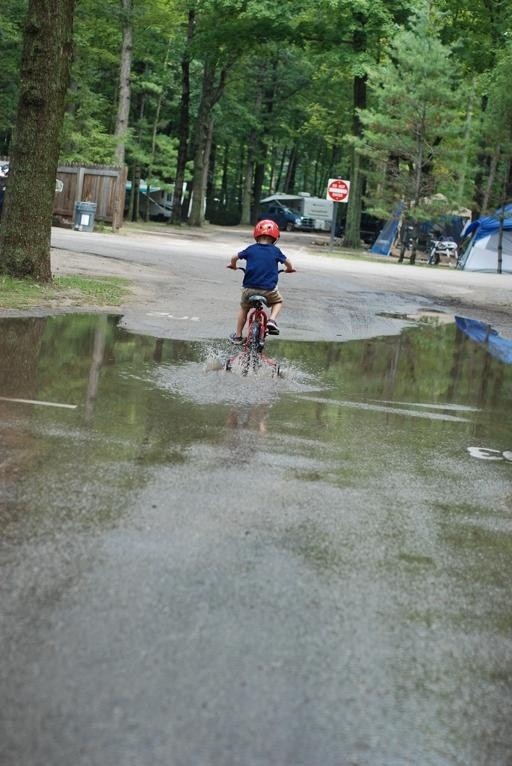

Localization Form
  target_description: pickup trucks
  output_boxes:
[256,203,316,232]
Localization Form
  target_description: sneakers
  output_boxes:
[227,332,243,345]
[266,318,280,335]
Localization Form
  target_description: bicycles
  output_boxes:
[226,265,296,379]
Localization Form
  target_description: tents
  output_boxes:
[369,193,472,267]
[454,204,511,274]
[455,315,511,364]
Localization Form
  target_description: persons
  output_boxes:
[228,219,294,344]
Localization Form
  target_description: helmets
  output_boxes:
[254,218,280,240]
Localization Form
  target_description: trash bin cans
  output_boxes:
[75,200,97,231]
